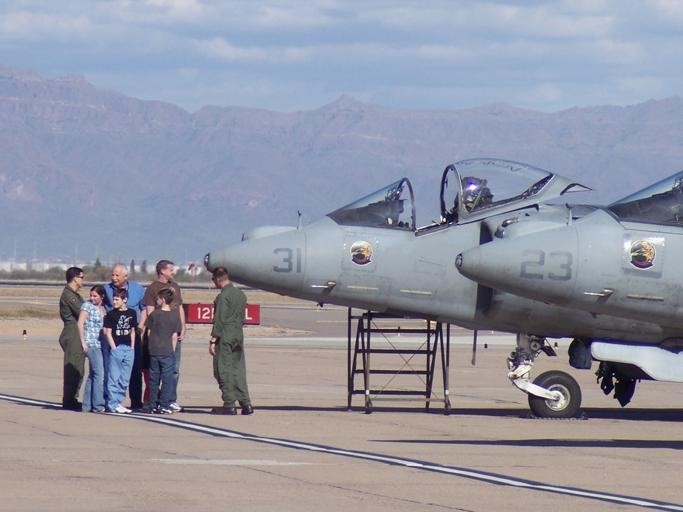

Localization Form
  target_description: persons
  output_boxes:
[207,267,252,415]
[141,260,185,413]
[100,263,145,409]
[100,288,136,414]
[76,285,105,413]
[140,288,181,414]
[58,266,85,409]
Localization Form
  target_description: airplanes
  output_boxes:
[203,156,683,418]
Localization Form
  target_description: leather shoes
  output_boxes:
[212,405,253,415]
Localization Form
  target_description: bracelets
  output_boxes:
[210,340,215,345]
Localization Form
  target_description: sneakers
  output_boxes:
[92,400,181,412]
